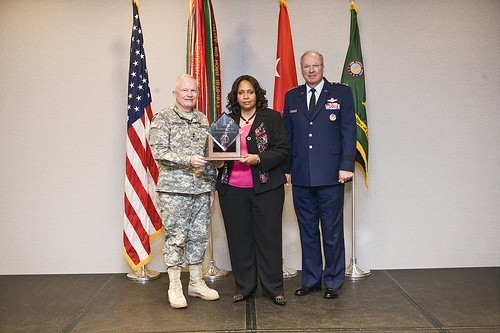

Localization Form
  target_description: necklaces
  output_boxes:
[240,111,257,124]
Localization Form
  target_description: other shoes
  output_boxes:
[273,295,287,306]
[232,294,247,302]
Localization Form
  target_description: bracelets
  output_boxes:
[255,155,261,165]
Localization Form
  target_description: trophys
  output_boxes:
[204,111,245,160]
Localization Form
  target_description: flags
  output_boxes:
[340,4,369,189]
[185,0,223,126]
[123,0,165,271]
[273,2,299,116]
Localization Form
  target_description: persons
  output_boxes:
[284,50,357,298]
[149,74,224,309]
[214,75,289,306]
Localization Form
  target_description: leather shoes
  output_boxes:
[322,288,343,299]
[294,287,322,296]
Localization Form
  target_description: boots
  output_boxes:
[188,264,220,300]
[167,266,188,308]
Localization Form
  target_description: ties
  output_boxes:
[309,88,316,119]
